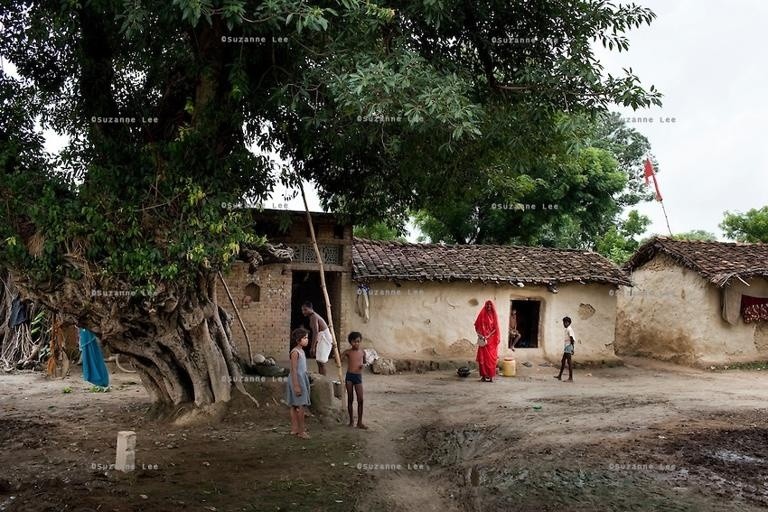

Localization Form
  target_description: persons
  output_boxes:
[299,300,332,376]
[510,308,521,352]
[474,300,500,385]
[284,324,311,442]
[553,316,576,383]
[332,332,368,429]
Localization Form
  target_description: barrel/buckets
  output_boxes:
[502,358,517,377]
